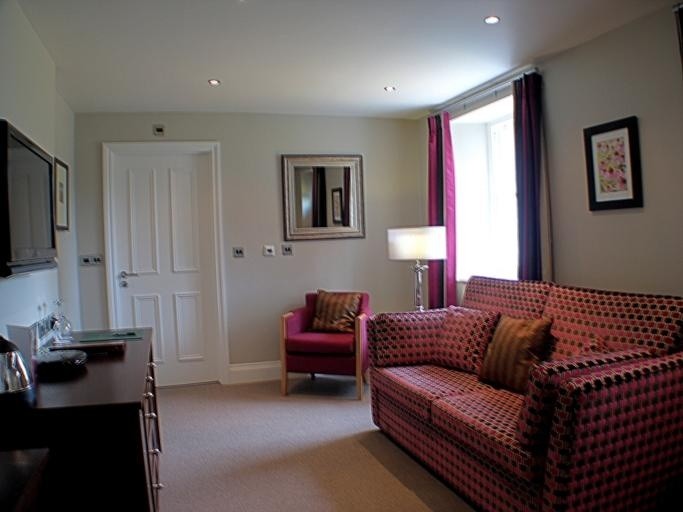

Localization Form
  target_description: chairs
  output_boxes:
[279,292,369,402]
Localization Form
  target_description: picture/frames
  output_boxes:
[53,156,70,231]
[581,114,643,212]
[331,188,344,224]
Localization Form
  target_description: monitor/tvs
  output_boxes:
[0,118,58,262]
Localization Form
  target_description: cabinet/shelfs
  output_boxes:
[32,325,160,509]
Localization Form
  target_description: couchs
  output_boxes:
[365,275,683,512]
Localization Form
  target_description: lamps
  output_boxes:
[386,226,447,312]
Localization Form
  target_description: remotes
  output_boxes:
[50,340,126,354]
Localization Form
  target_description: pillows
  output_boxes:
[310,289,360,334]
[433,304,608,395]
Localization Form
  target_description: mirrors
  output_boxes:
[279,152,365,243]
